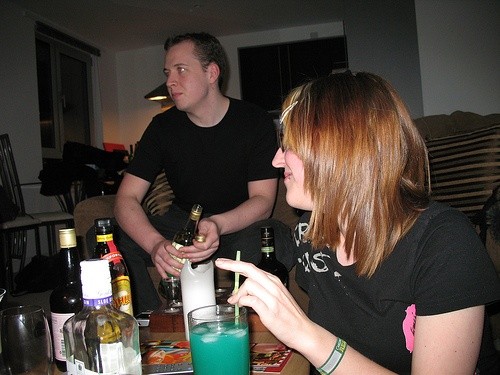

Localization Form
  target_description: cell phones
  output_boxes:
[135,310,152,327]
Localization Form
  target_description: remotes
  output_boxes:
[141,362,194,375]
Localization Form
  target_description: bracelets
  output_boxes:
[317,337,347,375]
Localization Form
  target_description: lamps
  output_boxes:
[145,81,170,101]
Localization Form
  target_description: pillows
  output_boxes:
[425,123,500,216]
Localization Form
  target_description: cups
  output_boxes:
[187,304,251,375]
[0,304,53,375]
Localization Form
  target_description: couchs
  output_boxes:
[73,110,500,314]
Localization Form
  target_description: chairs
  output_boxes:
[0,133,88,296]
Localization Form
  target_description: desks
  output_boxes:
[34,315,313,374]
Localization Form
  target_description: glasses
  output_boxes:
[279,125,284,153]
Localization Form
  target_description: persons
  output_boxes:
[86,33,298,316]
[215,69,500,375]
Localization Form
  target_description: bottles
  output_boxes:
[257,225,289,289]
[63,258,145,375]
[128,144,134,162]
[165,204,203,277]
[92,219,137,347]
[48,228,82,372]
